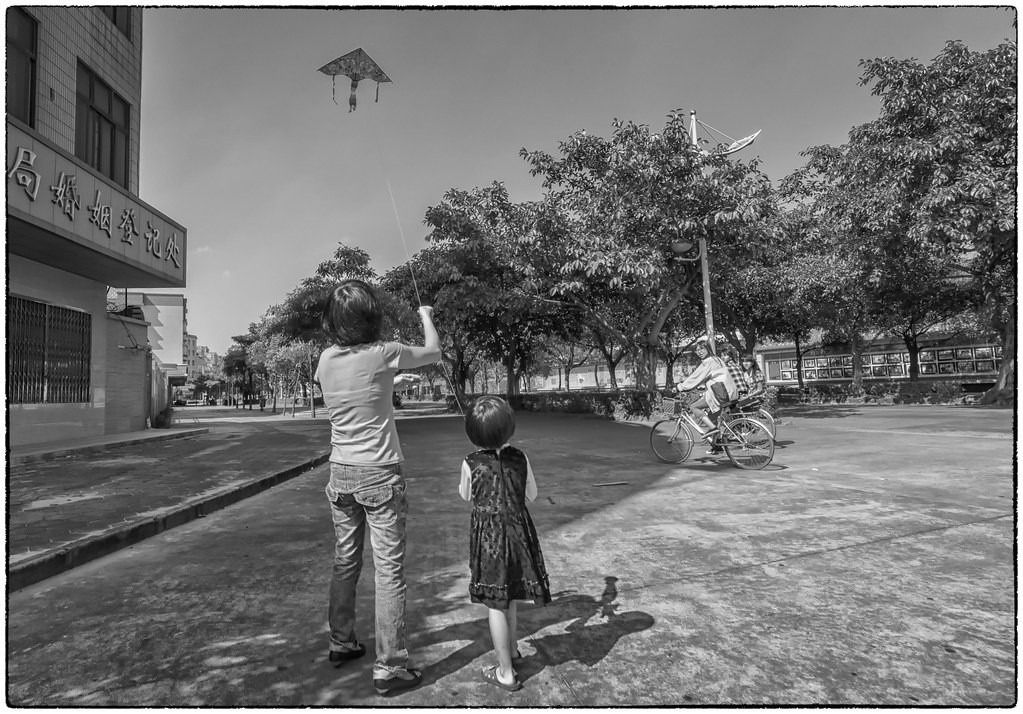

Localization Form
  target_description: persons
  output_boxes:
[316,280,442,695]
[672,340,767,454]
[459,396,552,691]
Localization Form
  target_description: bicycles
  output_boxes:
[651,383,776,471]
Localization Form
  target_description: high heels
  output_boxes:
[374,668,423,697]
[329,643,366,668]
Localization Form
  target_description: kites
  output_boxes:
[317,48,393,112]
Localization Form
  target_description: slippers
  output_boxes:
[511,649,524,665]
[481,667,522,690]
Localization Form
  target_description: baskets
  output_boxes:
[662,397,684,414]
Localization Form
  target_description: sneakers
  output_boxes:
[706,447,724,454]
[701,427,720,439]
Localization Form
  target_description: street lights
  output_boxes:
[670,110,762,356]
